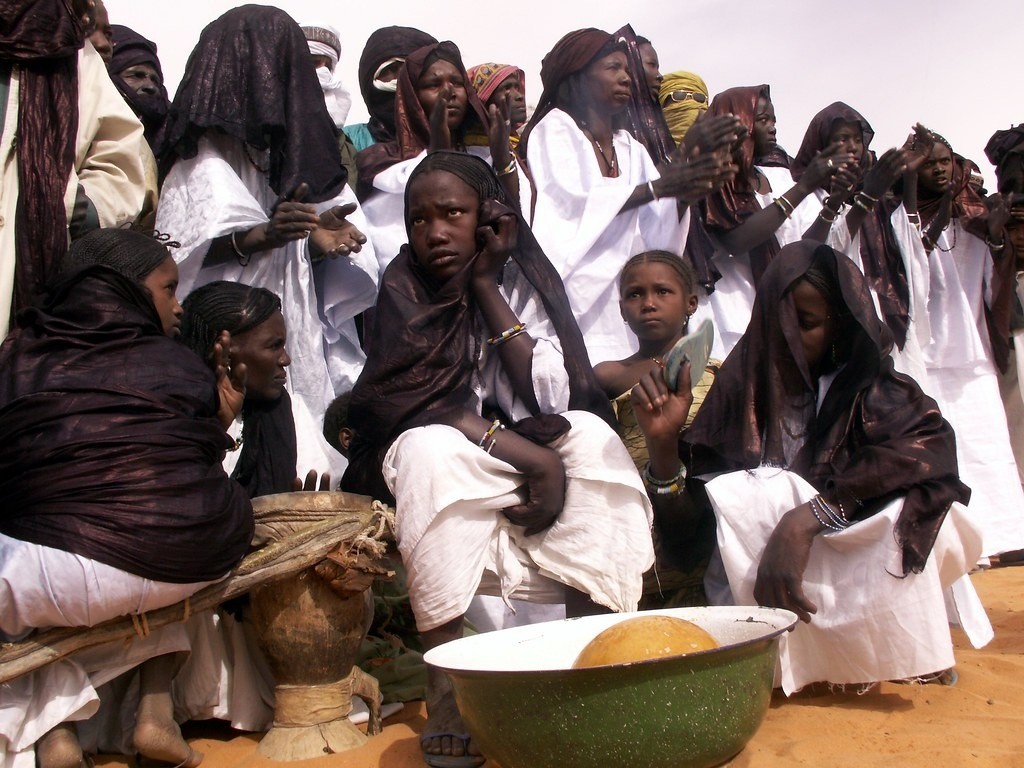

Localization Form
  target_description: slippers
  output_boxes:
[420,731,485,768]
[660,319,717,389]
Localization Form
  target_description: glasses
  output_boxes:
[668,89,708,104]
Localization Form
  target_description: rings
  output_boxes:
[338,244,344,251]
[827,160,832,167]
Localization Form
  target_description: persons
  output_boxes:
[0,0,1024,768]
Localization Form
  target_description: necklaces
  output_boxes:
[932,210,956,252]
[243,141,270,173]
[755,174,761,192]
[639,351,659,364]
[225,407,245,452]
[584,125,615,176]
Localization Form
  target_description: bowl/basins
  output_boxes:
[423,605,800,768]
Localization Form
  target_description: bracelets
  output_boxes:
[985,234,1003,251]
[809,495,849,531]
[773,195,794,219]
[478,419,505,453]
[923,233,938,252]
[642,457,687,499]
[492,151,518,176]
[311,255,323,262]
[486,322,529,346]
[229,232,246,259]
[906,214,920,227]
[819,196,846,223]
[853,191,878,213]
[648,180,659,202]
